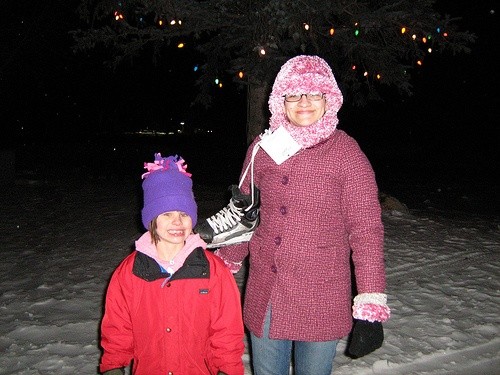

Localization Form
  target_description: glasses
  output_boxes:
[282,91,326,102]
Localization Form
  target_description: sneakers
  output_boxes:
[192,183,261,249]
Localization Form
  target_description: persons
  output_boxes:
[99,153,245,375]
[213,55,390,375]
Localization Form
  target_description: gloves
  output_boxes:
[343,303,391,360]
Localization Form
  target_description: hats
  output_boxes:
[267,55,344,149]
[141,152,197,230]
